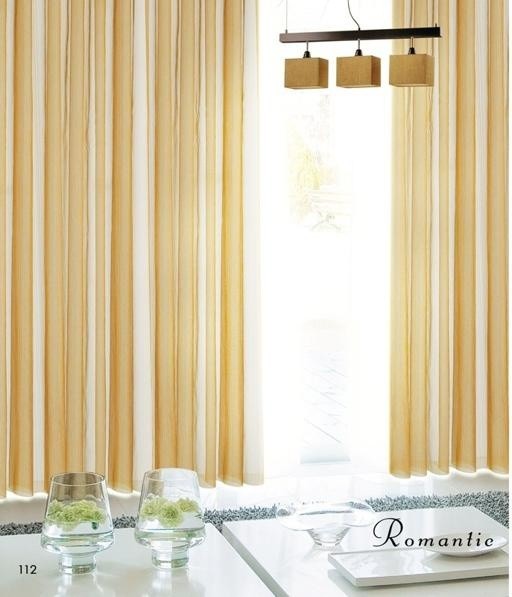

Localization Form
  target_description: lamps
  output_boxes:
[279,0,441,88]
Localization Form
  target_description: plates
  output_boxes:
[329,531,508,589]
[275,500,376,545]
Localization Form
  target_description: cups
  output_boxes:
[40,468,207,576]
